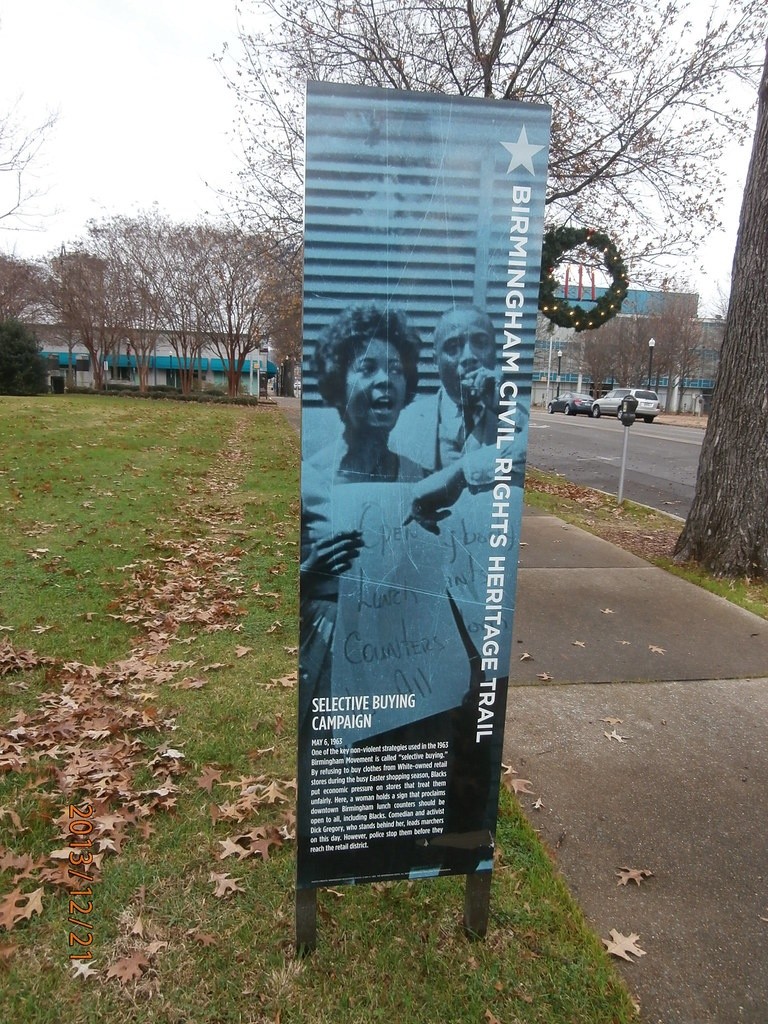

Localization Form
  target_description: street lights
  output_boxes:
[557,350,562,397]
[169,351,173,386]
[647,338,655,390]
[280,362,284,396]
[276,367,279,396]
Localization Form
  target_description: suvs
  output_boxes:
[591,388,660,423]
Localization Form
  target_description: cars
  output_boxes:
[547,392,594,417]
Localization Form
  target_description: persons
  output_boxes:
[407,429,527,536]
[387,298,527,472]
[300,300,486,752]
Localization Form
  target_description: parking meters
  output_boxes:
[618,395,638,502]
